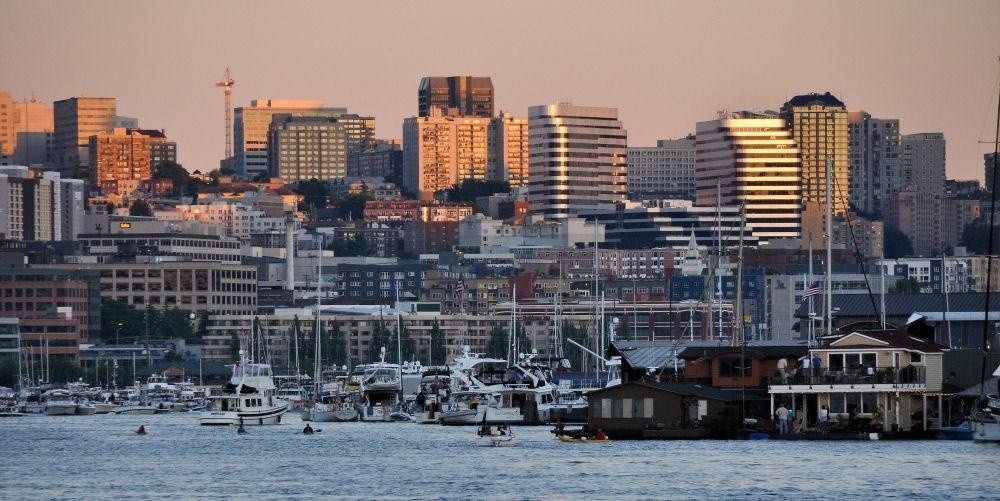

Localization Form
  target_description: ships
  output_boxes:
[199,240,402,426]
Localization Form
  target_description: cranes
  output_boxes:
[214,68,235,160]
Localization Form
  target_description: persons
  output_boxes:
[819,405,828,433]
[777,352,822,385]
[776,403,808,436]
[570,426,588,439]
[477,425,508,437]
[591,426,606,440]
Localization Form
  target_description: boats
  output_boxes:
[926,422,973,441]
[475,422,517,447]
[550,422,610,442]
[642,427,723,441]
[0,323,195,424]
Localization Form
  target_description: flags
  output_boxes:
[455,272,464,299]
[804,280,820,298]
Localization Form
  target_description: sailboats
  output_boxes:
[963,95,999,442]
[404,218,607,427]
